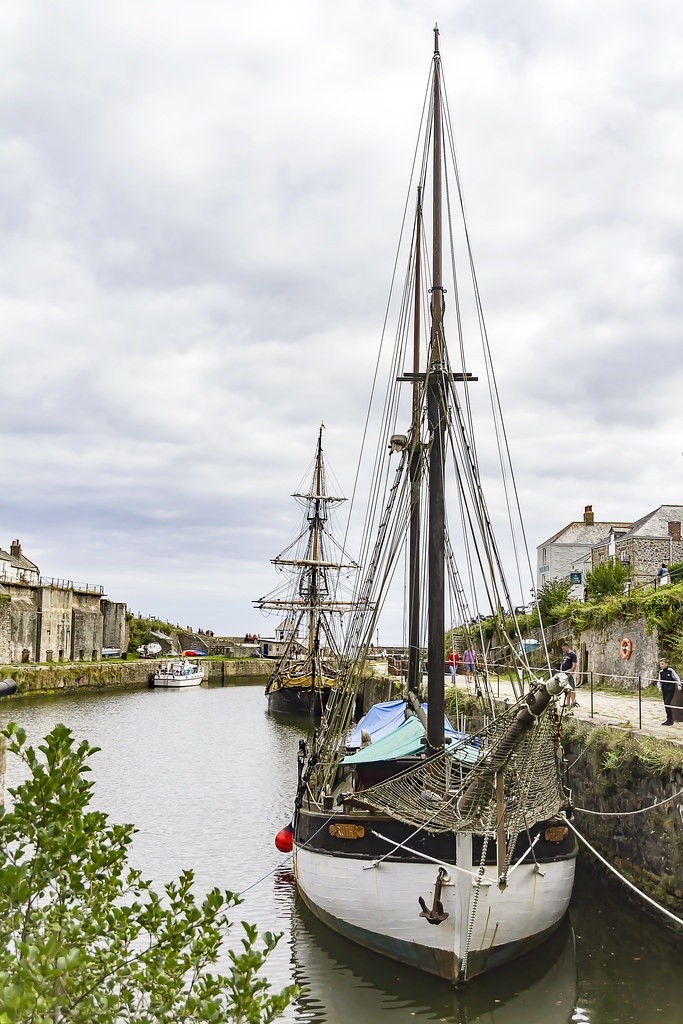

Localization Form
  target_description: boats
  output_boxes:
[167,650,178,657]
[151,655,206,687]
[136,641,162,656]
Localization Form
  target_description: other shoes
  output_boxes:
[661,718,674,726]
[561,704,568,708]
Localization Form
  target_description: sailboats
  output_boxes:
[249,423,367,723]
[275,18,581,981]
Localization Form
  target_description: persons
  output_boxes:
[244,632,260,644]
[657,563,668,579]
[462,644,476,683]
[558,643,576,707]
[656,658,682,725]
[448,647,459,684]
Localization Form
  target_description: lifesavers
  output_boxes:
[149,673,157,689]
[621,638,632,659]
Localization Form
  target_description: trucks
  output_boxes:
[102,647,122,658]
